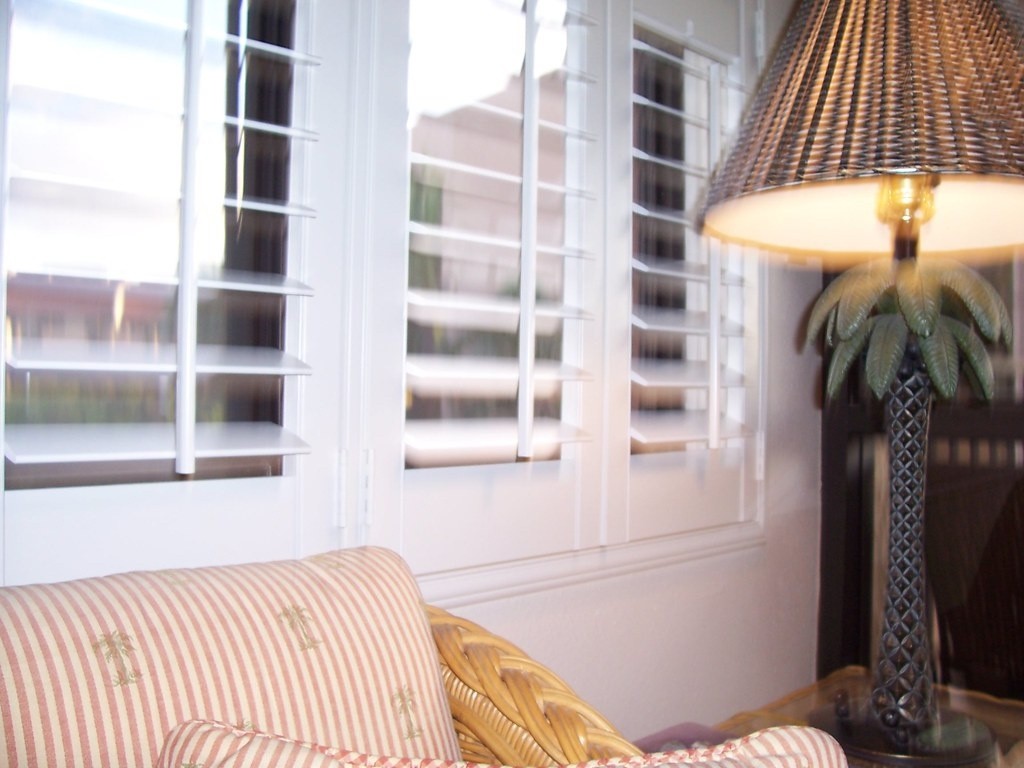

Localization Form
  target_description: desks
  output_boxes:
[714,666,1024,768]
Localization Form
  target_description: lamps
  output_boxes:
[694,0,1024,768]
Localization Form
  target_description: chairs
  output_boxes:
[426,603,642,764]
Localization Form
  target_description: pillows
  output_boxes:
[154,720,851,768]
[0,544,461,768]
